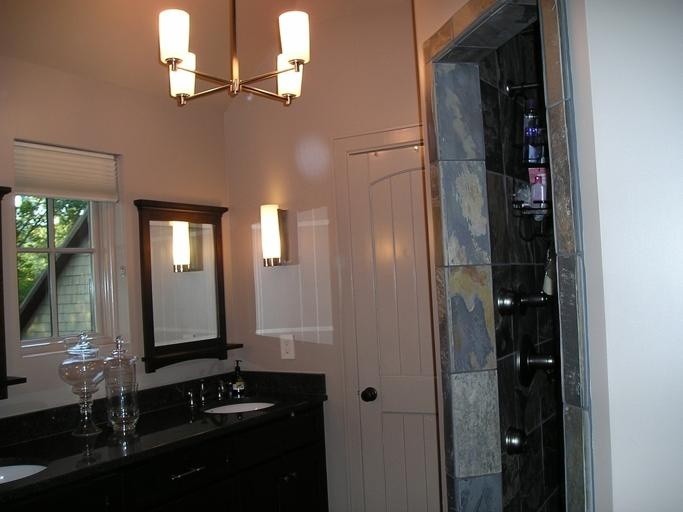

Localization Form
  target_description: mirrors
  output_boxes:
[133,200,227,374]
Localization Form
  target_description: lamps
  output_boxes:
[260,203,290,268]
[158,1,310,106]
[168,221,201,272]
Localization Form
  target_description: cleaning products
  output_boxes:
[530,176,545,206]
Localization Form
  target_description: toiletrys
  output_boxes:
[232,358,249,399]
[529,166,548,184]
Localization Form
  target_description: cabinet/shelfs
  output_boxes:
[0,395,329,511]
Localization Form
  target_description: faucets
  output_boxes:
[198,378,208,407]
[216,378,228,401]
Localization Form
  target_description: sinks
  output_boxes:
[0,463,50,485]
[203,398,276,415]
